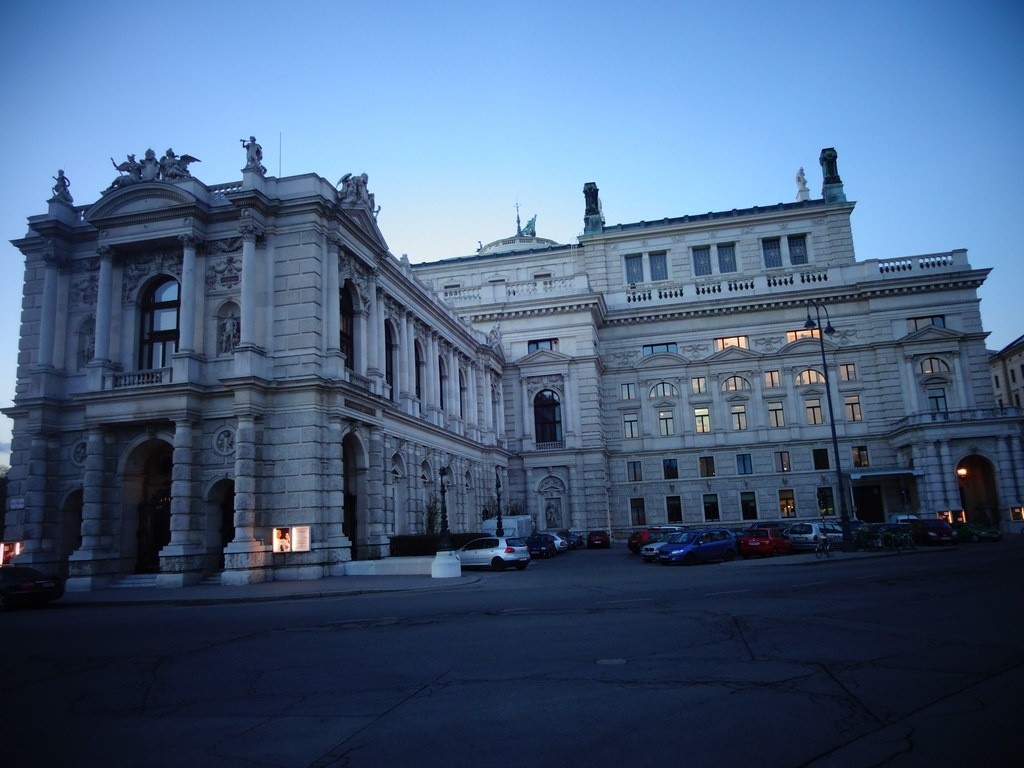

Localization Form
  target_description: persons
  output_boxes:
[240,136,262,167]
[51,170,72,202]
[110,149,191,183]
[814,527,832,558]
[221,312,237,353]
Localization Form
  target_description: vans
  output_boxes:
[788,520,843,553]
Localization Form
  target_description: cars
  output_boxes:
[0,564,64,614]
[629,527,674,555]
[524,531,568,556]
[859,514,959,549]
[457,535,531,572]
[740,527,798,559]
[640,531,693,564]
[587,530,610,549]
[655,526,738,568]
[553,529,584,549]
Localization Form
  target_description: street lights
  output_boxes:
[804,298,853,543]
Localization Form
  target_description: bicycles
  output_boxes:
[814,533,836,559]
